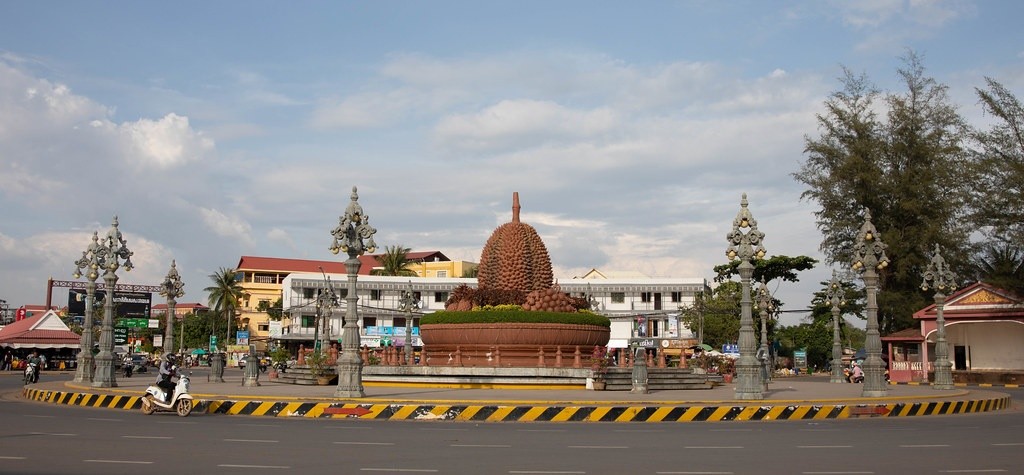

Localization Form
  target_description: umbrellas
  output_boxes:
[192,349,206,354]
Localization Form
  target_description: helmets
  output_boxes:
[853,363,858,367]
[166,353,176,364]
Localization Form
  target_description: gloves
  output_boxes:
[170,373,176,377]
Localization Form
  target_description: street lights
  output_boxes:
[726,192,768,400]
[327,185,378,399]
[316,274,340,357]
[396,279,420,362]
[752,274,775,383]
[72,230,100,383]
[919,243,961,391]
[850,207,891,397]
[92,213,134,387]
[824,269,848,383]
[159,259,186,361]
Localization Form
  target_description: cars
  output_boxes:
[238,352,272,370]
[273,359,297,369]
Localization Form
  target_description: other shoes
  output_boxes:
[166,400,171,405]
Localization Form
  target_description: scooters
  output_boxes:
[260,362,266,374]
[140,365,194,417]
[23,356,41,385]
[120,360,135,378]
[845,367,864,383]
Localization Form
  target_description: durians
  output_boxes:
[477,192,554,292]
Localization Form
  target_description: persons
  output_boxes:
[4,351,13,371]
[829,365,832,375]
[260,356,267,373]
[156,353,190,405]
[850,363,860,383]
[289,356,296,367]
[122,353,134,377]
[185,355,191,368]
[27,351,46,383]
